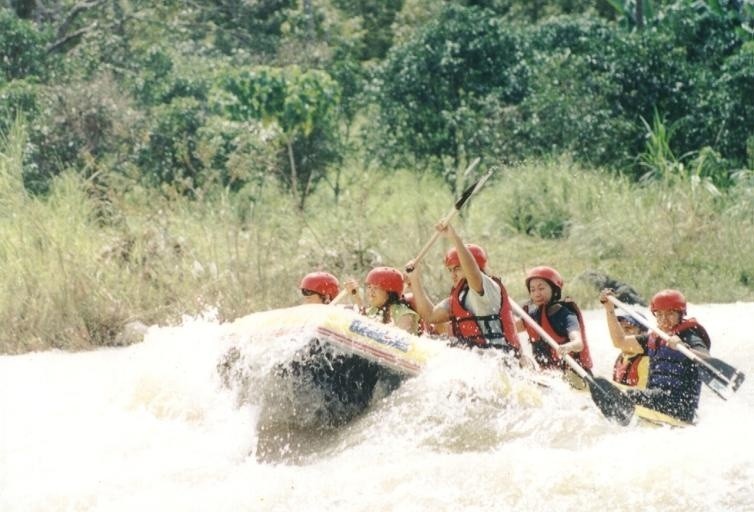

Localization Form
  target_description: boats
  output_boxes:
[217,302,702,467]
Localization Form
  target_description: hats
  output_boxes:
[616,312,650,332]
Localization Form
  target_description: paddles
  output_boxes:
[600,288,746,402]
[507,297,636,426]
[406,169,493,272]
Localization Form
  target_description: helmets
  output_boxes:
[365,266,404,298]
[445,244,488,270]
[300,272,339,300]
[650,289,687,317]
[525,267,565,290]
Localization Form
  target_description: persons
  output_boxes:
[298,271,362,305]
[405,220,521,362]
[599,288,711,425]
[515,266,593,369]
[612,311,650,389]
[366,267,419,336]
